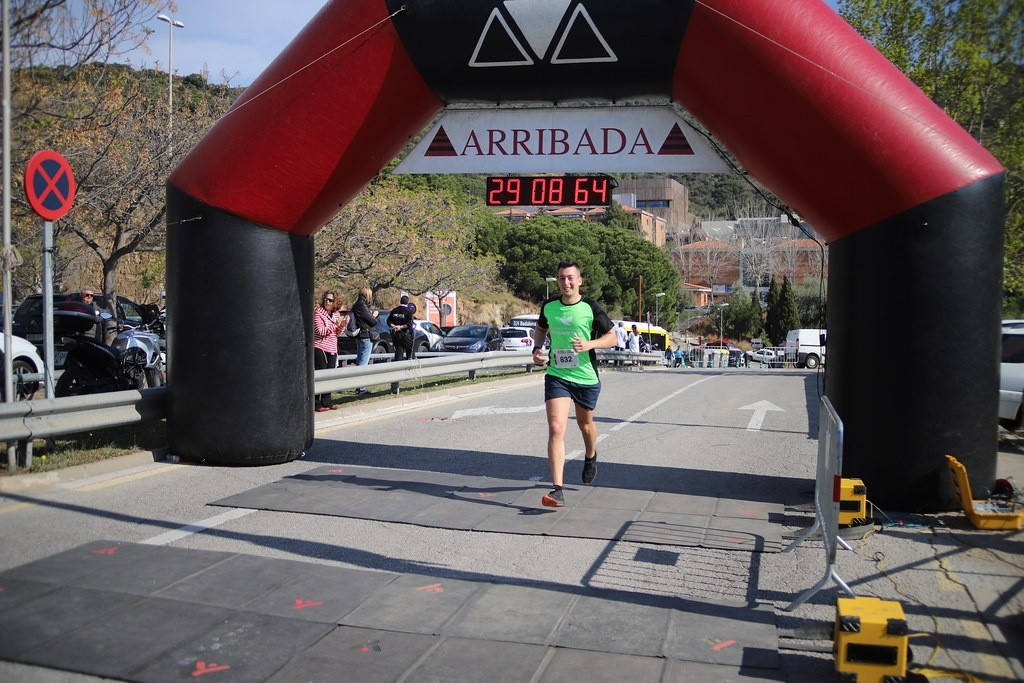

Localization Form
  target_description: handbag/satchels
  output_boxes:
[370,331,380,341]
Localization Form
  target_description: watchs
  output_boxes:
[532,346,541,354]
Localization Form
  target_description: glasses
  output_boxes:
[84,293,94,296]
[324,298,334,301]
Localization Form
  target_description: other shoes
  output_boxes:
[356,389,367,395]
[317,406,328,411]
[327,404,337,408]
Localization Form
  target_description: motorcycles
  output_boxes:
[55,293,166,397]
[639,337,658,366]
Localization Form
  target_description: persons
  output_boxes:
[600,320,639,366]
[531,258,617,507]
[673,345,684,368]
[81,285,104,338]
[664,346,674,367]
[386,296,414,360]
[743,352,748,367]
[314,288,351,409]
[351,285,380,395]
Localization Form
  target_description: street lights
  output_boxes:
[656,293,665,326]
[546,277,557,299]
[721,303,729,349]
[158,14,184,158]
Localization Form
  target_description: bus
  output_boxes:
[507,312,669,366]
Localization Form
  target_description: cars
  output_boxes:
[411,320,551,355]
[676,304,714,315]
[705,341,743,365]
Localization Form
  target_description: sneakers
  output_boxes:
[582,450,597,483]
[543,490,564,506]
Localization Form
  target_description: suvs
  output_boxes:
[334,310,430,366]
[0,289,144,404]
[997,320,1024,433]
[745,346,776,363]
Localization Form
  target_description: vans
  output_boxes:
[751,338,763,349]
[785,328,828,369]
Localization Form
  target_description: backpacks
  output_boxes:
[345,309,359,336]
